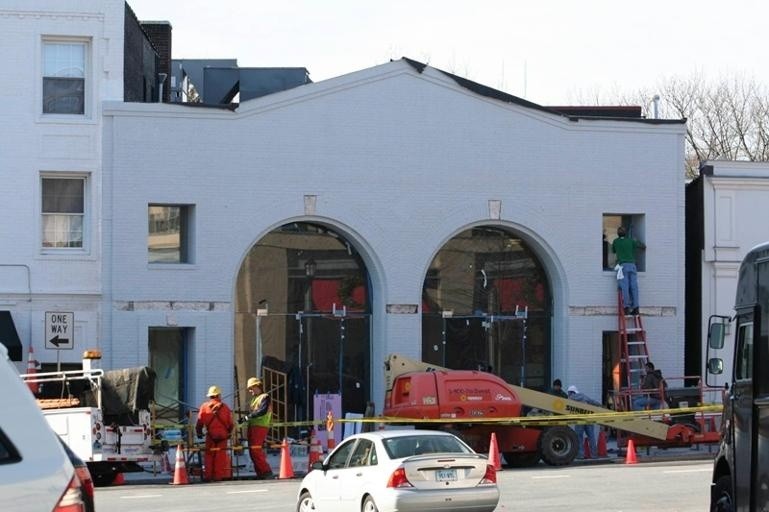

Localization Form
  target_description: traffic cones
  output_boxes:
[22,345,40,397]
[111,471,130,487]
[161,454,173,474]
[483,431,504,473]
[168,442,193,487]
[277,427,326,480]
[580,429,641,466]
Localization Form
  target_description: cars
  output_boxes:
[54,430,95,512]
[294,428,503,512]
[0,338,85,512]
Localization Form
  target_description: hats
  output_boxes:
[553,378,561,386]
[568,386,578,393]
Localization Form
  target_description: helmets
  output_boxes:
[247,377,262,388]
[207,386,221,397]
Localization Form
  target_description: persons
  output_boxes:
[633,362,668,409]
[567,385,601,459]
[548,379,568,416]
[612,227,646,318]
[237,377,274,479]
[196,385,234,480]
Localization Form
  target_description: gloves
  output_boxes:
[238,416,247,423]
[196,425,205,439]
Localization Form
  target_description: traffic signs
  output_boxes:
[44,311,74,348]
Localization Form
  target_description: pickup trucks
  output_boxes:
[16,366,161,488]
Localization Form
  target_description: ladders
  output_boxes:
[617,287,650,411]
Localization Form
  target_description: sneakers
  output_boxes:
[255,472,272,480]
[625,307,639,314]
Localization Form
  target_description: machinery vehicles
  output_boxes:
[376,350,724,469]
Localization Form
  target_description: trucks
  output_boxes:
[703,240,769,512]
